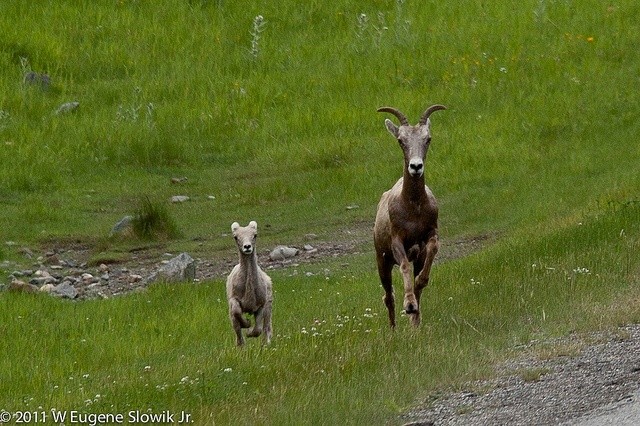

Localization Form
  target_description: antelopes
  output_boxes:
[373,103,447,331]
[225,220,274,349]
